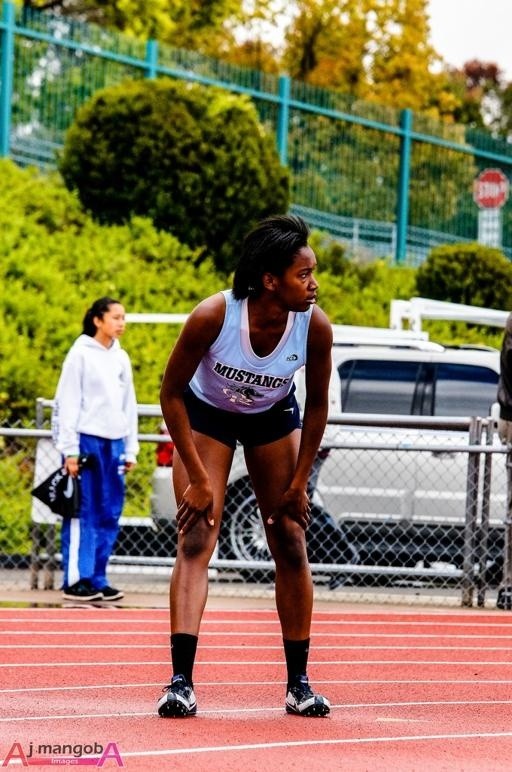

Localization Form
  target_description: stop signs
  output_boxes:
[473,168,508,209]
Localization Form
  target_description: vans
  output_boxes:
[152,347,512,586]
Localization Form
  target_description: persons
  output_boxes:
[156,212,334,718]
[497,312,512,610]
[50,296,140,601]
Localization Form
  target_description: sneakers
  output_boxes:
[63,580,124,600]
[497,587,512,609]
[285,683,330,716]
[157,674,196,718]
